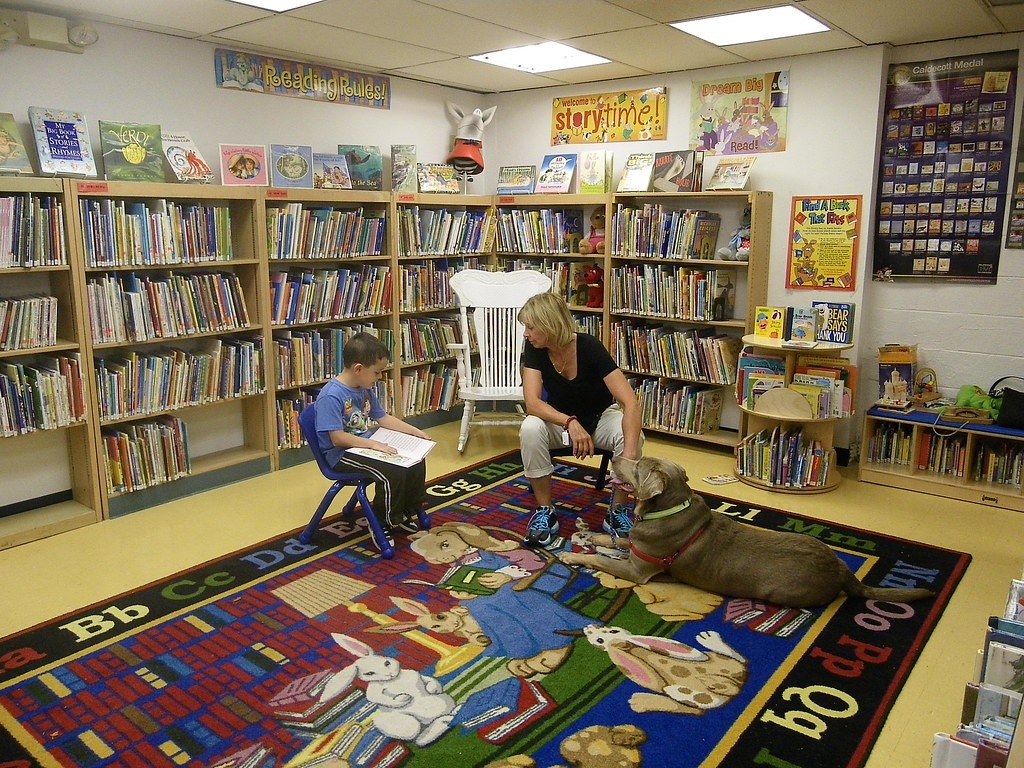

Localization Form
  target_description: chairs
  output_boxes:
[529,384,614,492]
[446,269,552,452]
[298,403,430,559]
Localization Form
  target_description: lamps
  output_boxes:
[0,8,99,54]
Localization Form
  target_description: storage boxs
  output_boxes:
[879,343,918,400]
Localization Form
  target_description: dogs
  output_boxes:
[555,455,936,609]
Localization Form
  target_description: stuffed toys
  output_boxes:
[582,263,604,308]
[579,206,605,254]
[718,204,751,261]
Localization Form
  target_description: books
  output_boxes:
[734,300,855,419]
[270,257,594,326]
[0,105,757,192]
[93,334,267,421]
[0,292,59,351]
[265,203,584,259]
[0,192,233,267]
[344,427,437,468]
[737,423,829,488]
[930,578,1024,768]
[273,313,482,449]
[609,203,744,435]
[87,270,250,345]
[867,422,1024,489]
[101,414,192,494]
[0,352,88,438]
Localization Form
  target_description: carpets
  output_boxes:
[0,450,972,767]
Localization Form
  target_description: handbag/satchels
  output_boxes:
[988,376,1024,431]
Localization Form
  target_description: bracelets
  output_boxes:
[564,415,576,429]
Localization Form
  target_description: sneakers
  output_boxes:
[398,509,419,533]
[523,505,560,547]
[367,522,395,550]
[601,502,634,538]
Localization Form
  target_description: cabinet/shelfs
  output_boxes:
[608,190,773,448]
[857,409,1024,513]
[493,193,609,351]
[733,333,854,495]
[0,178,493,550]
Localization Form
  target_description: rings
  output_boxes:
[552,359,566,374]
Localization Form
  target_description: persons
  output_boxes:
[314,332,432,550]
[517,293,646,547]
